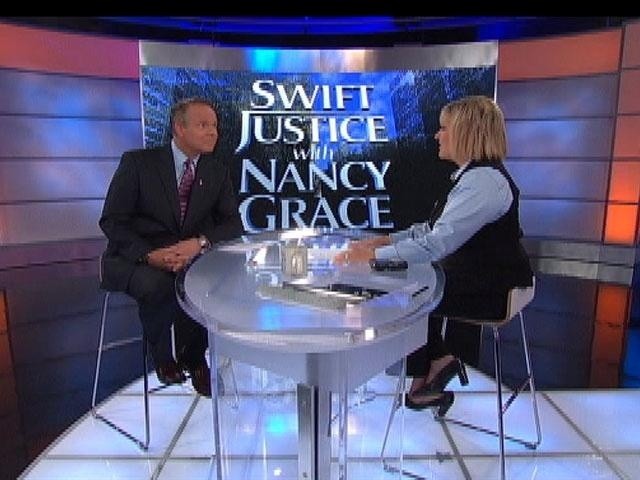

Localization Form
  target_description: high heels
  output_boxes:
[399,358,469,420]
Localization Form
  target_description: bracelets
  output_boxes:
[198,234,209,255]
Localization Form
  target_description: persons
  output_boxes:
[98,92,243,397]
[330,93,535,420]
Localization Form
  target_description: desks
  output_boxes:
[175,225,445,480]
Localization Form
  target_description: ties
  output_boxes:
[177,159,196,225]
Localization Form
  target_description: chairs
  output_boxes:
[89,236,210,450]
[380,268,542,480]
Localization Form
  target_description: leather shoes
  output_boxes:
[155,344,211,399]
